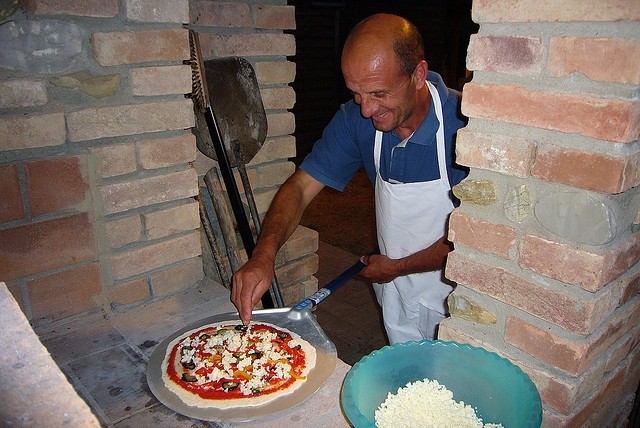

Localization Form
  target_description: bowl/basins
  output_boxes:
[341,339,542,428]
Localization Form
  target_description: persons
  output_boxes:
[231,13,468,346]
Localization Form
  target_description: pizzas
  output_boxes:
[160,319,317,412]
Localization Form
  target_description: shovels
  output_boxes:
[183,56,284,307]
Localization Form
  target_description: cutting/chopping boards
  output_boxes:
[144,254,371,424]
